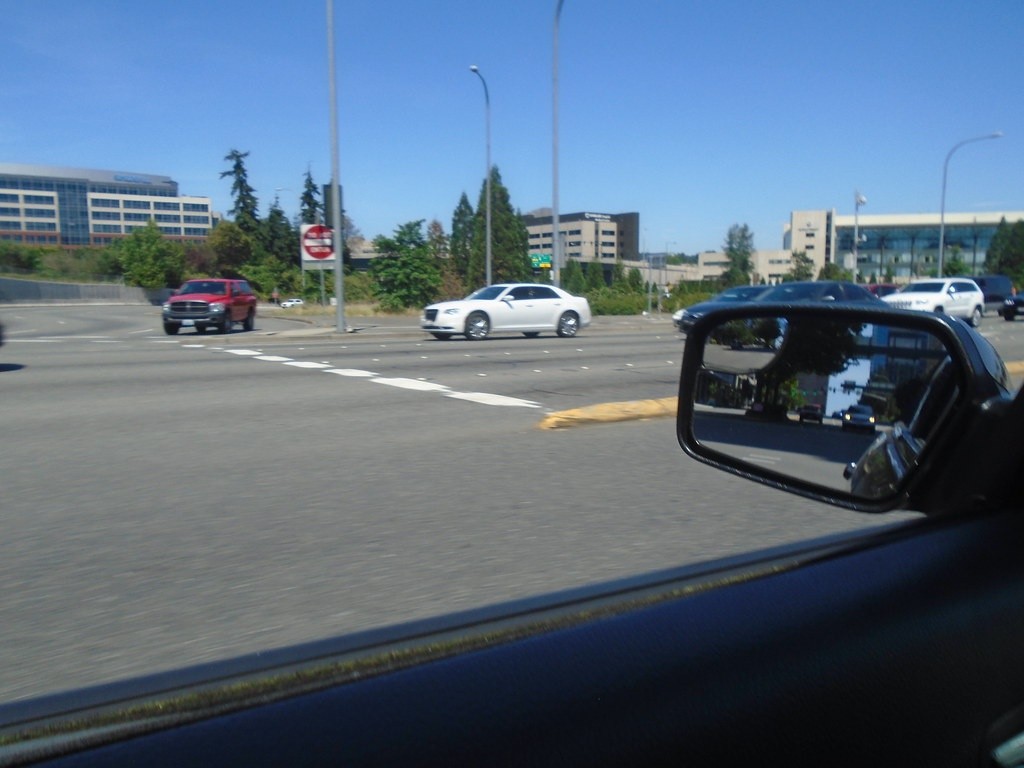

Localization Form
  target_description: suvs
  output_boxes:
[879,278,985,327]
[162,279,256,335]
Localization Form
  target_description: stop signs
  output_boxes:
[300,224,339,261]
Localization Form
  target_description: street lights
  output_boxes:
[936,132,1001,278]
[469,65,492,288]
[852,195,866,279]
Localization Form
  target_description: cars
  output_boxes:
[281,299,303,308]
[799,403,821,424]
[841,405,877,434]
[421,282,590,341]
[971,274,1015,311]
[673,282,896,349]
